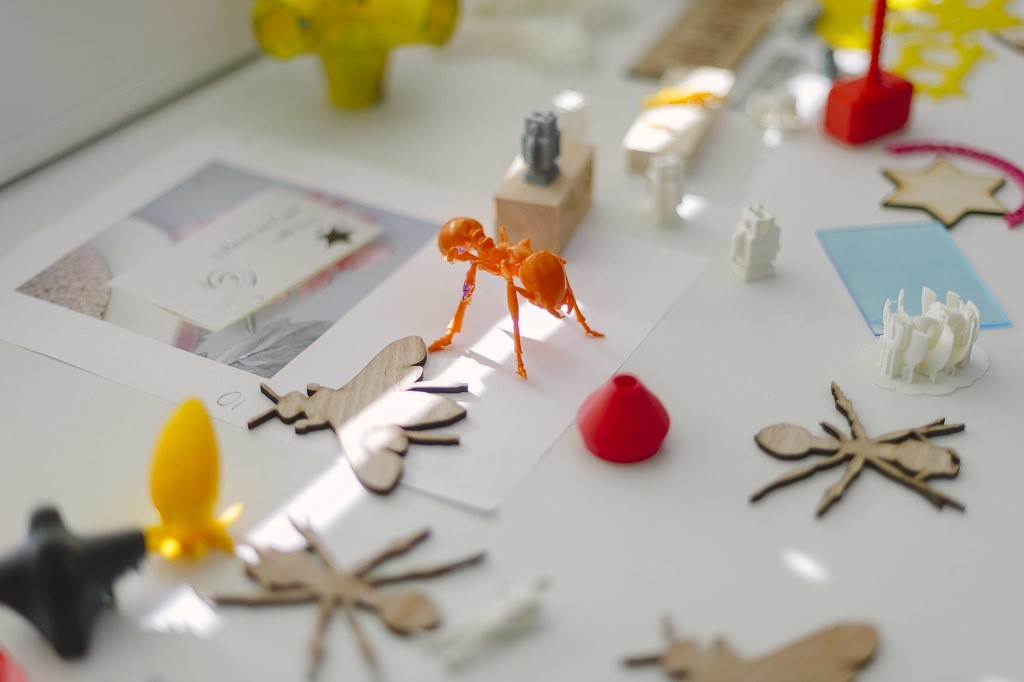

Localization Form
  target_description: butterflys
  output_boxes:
[142,395,241,573]
[625,616,876,682]
[249,336,467,494]
